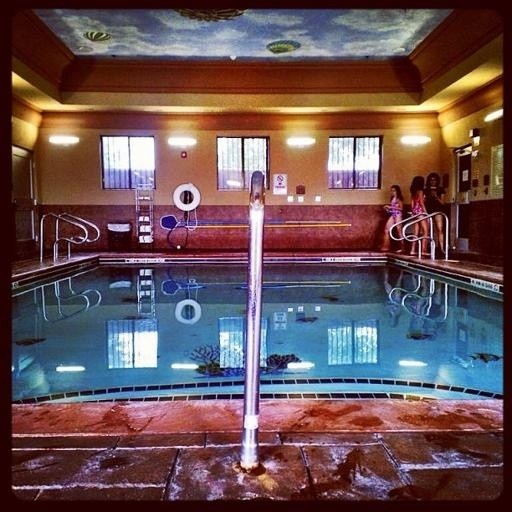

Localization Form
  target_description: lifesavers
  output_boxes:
[172,183,201,212]
[174,299,202,325]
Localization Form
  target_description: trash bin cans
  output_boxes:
[106,222,133,252]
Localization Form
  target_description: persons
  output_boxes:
[383,267,403,327]
[380,185,407,253]
[410,176,431,255]
[423,173,446,254]
[407,273,441,340]
